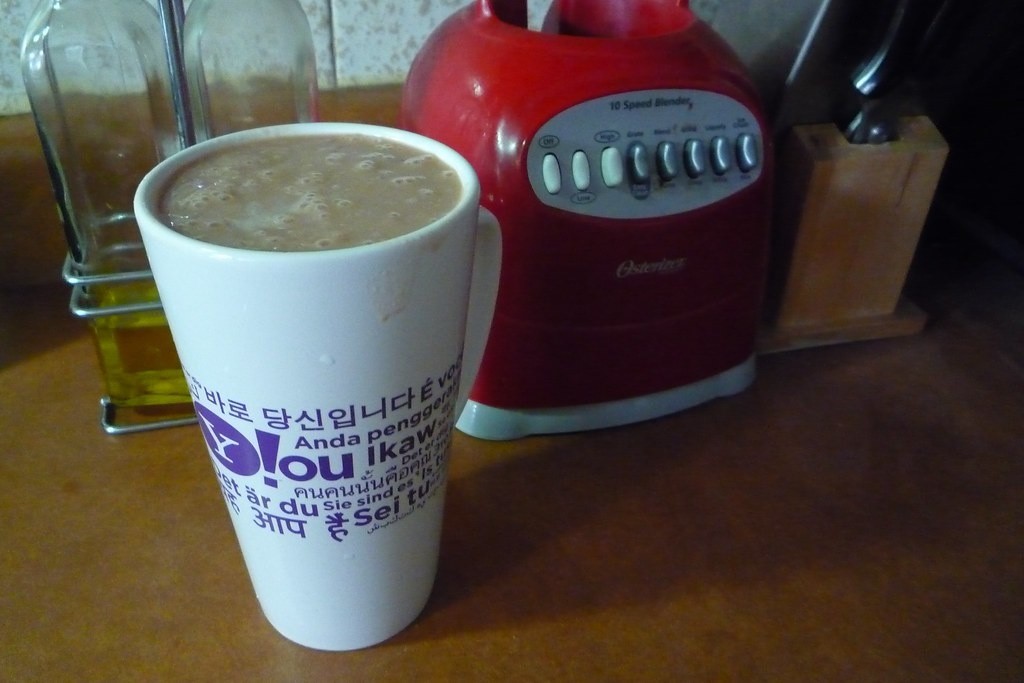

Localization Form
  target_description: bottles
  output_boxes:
[19,0,201,425]
[182,0,319,144]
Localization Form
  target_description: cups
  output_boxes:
[130,121,503,652]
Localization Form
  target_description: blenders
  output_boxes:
[392,1,772,440]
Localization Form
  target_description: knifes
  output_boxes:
[776,0,966,157]
